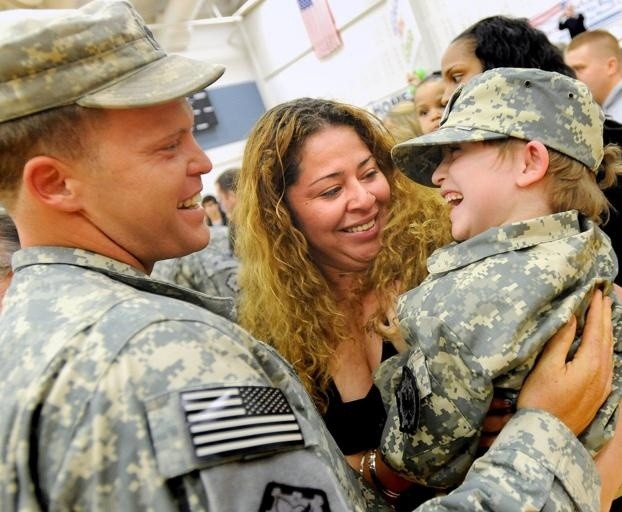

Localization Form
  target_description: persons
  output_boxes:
[1,213,21,296]
[152,169,245,320]
[233,98,622,512]
[374,70,621,492]
[413,16,620,286]
[0,0,612,512]
[559,3,588,40]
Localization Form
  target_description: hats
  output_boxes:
[388,65,608,188]
[0,3,228,126]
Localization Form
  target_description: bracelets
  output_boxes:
[360,451,367,478]
[368,448,399,499]
